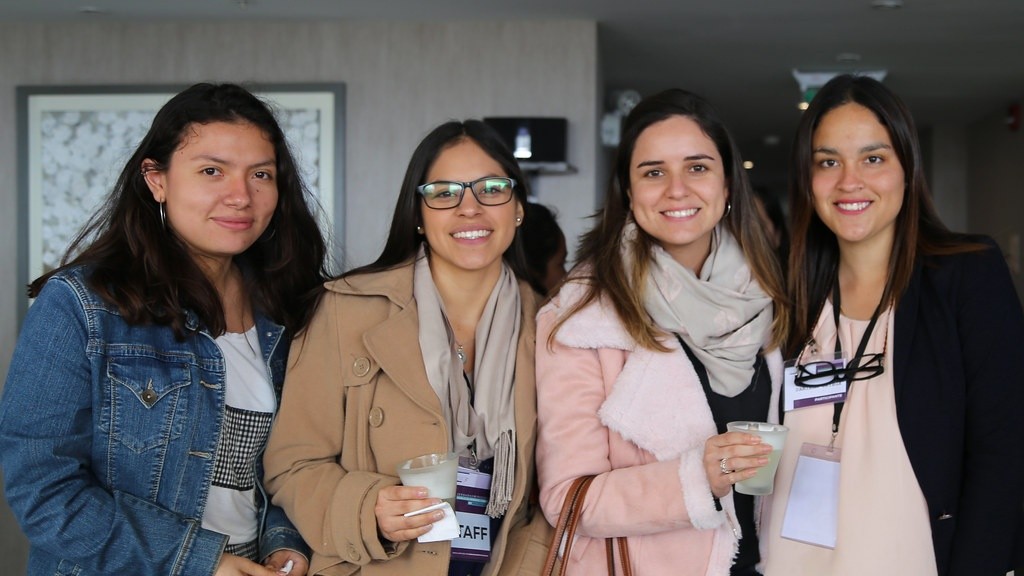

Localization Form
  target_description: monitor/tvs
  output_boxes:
[483,117,567,162]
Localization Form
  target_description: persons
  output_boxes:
[2,75,1023,576]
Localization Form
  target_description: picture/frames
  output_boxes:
[12,82,347,354]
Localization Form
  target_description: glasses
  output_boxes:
[415,175,518,211]
[793,352,885,387]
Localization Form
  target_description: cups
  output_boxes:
[398,451,458,519]
[727,421,789,495]
